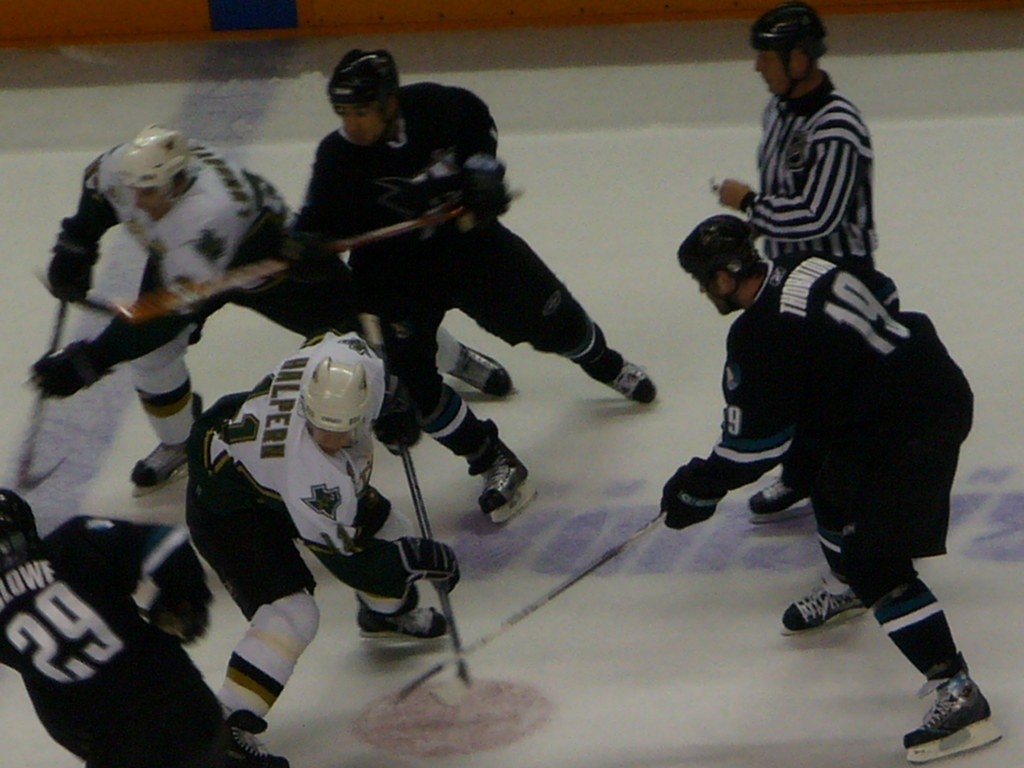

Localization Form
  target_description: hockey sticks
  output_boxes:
[37,182,506,321]
[392,433,475,689]
[15,273,79,489]
[383,512,661,704]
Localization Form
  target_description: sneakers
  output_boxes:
[468,436,537,522]
[356,591,450,648]
[221,709,290,768]
[747,477,814,525]
[449,343,518,401]
[780,566,864,635]
[582,349,656,404]
[130,434,191,497]
[902,650,1002,763]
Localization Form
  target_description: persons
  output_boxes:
[662,215,992,749]
[0,487,233,768]
[31,124,513,491]
[718,2,875,515]
[187,332,462,768]
[279,47,656,514]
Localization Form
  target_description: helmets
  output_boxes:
[0,487,43,560]
[748,2,830,61]
[679,213,755,298]
[324,47,400,108]
[117,124,190,195]
[297,335,386,448]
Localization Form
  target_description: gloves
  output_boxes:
[47,217,100,302]
[28,339,112,400]
[147,585,214,644]
[396,534,462,593]
[460,170,512,224]
[658,457,729,530]
[274,221,334,287]
